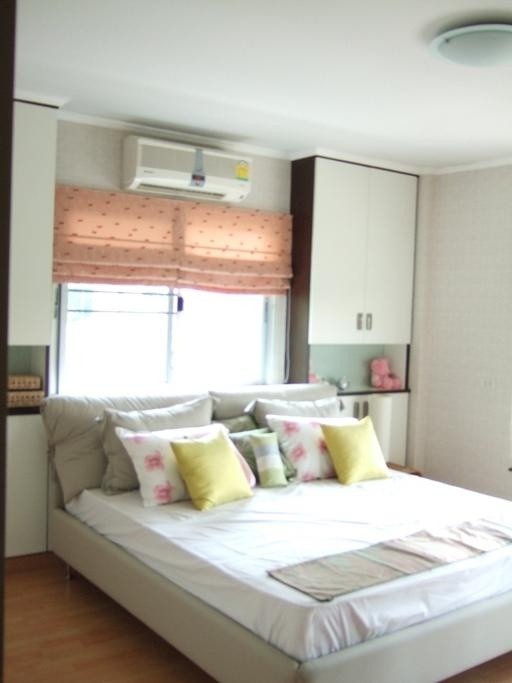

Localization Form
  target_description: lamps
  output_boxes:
[424,22,512,69]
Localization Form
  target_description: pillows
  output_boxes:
[170,426,253,512]
[99,392,215,498]
[216,414,258,434]
[320,415,391,486]
[245,431,287,489]
[265,413,358,483]
[114,425,257,507]
[245,394,341,429]
[229,425,298,482]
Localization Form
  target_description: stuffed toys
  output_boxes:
[371,357,401,391]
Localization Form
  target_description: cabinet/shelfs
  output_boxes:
[288,153,420,467]
[1,95,62,563]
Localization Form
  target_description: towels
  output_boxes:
[266,515,509,604]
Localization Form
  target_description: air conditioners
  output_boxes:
[121,133,257,204]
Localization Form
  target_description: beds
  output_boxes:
[37,378,510,682]
